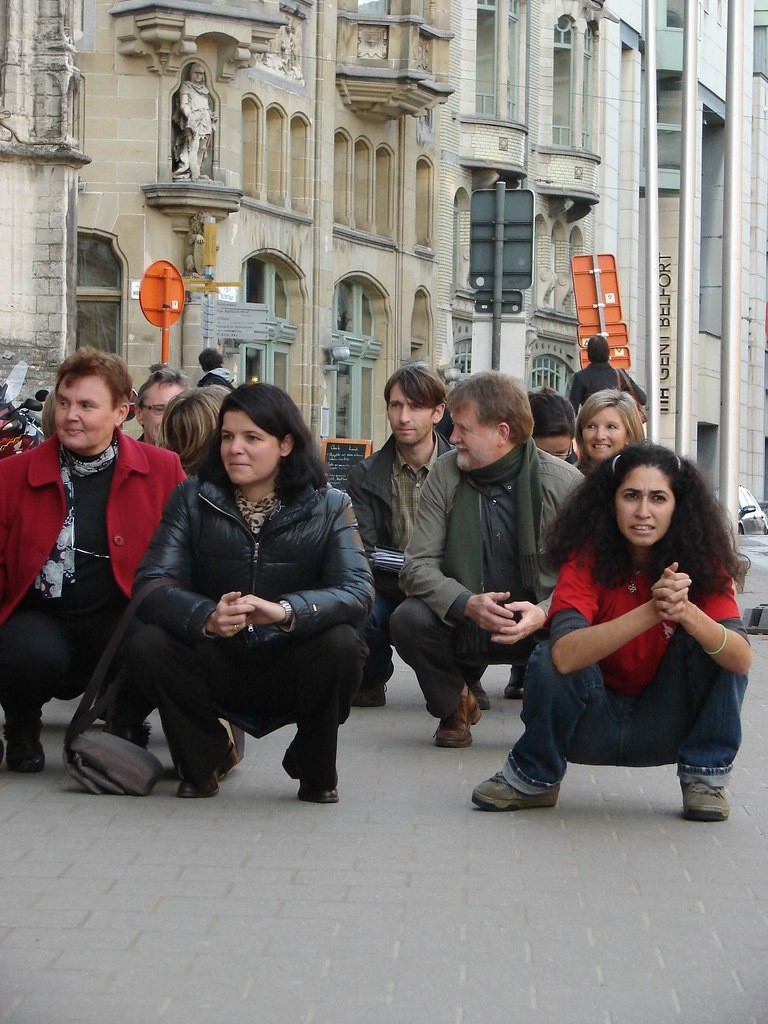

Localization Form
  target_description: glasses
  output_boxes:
[554,442,573,458]
[142,403,166,415]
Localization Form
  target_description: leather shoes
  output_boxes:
[471,680,491,711]
[434,685,482,748]
[298,779,339,803]
[111,719,151,752]
[176,742,239,798]
[504,679,524,699]
[6,718,44,772]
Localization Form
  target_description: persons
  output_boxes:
[0,334,752,819]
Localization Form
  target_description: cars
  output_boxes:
[738,485,768,535]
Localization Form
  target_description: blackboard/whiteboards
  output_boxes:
[321,438,373,491]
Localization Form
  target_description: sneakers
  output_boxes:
[680,777,730,822]
[471,770,560,812]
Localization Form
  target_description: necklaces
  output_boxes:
[627,571,642,593]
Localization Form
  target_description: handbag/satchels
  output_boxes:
[615,367,648,423]
[62,726,163,796]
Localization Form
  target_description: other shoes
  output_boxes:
[351,678,386,707]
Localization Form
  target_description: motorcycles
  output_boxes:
[0,360,138,460]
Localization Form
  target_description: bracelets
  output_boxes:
[689,606,699,635]
[278,600,292,623]
[704,624,727,655]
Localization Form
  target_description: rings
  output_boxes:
[233,624,239,631]
